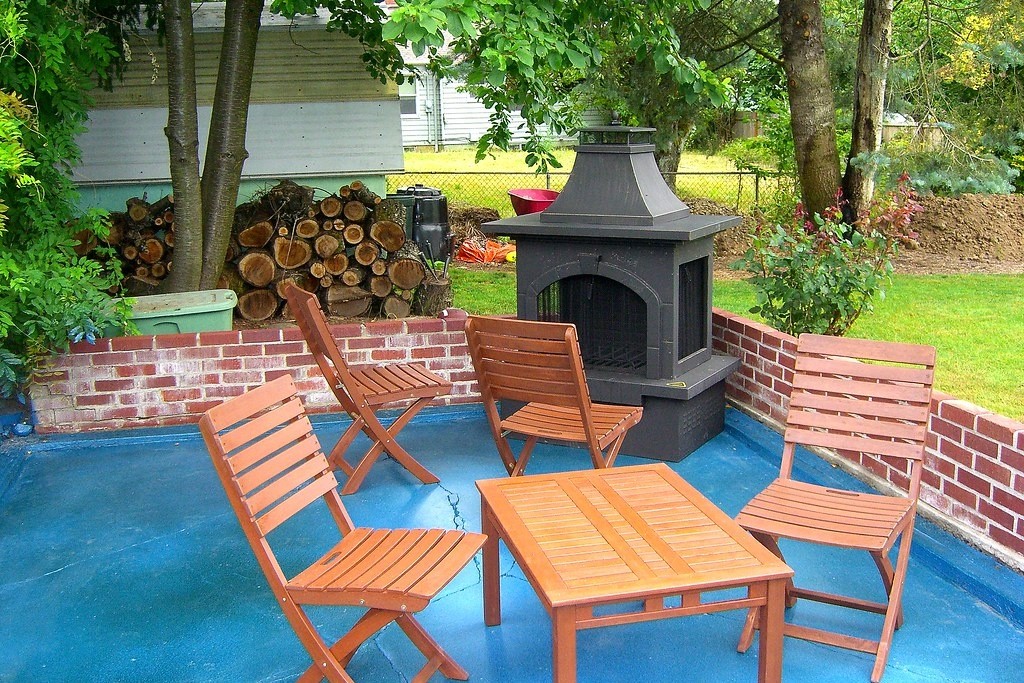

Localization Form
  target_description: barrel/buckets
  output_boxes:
[386,184,457,263]
[507,188,560,216]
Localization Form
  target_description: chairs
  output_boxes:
[197,373,488,683]
[281,279,455,495]
[462,315,643,476]
[733,333,936,683]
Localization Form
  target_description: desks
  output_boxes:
[475,462,796,683]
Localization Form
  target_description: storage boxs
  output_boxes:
[95,288,239,339]
